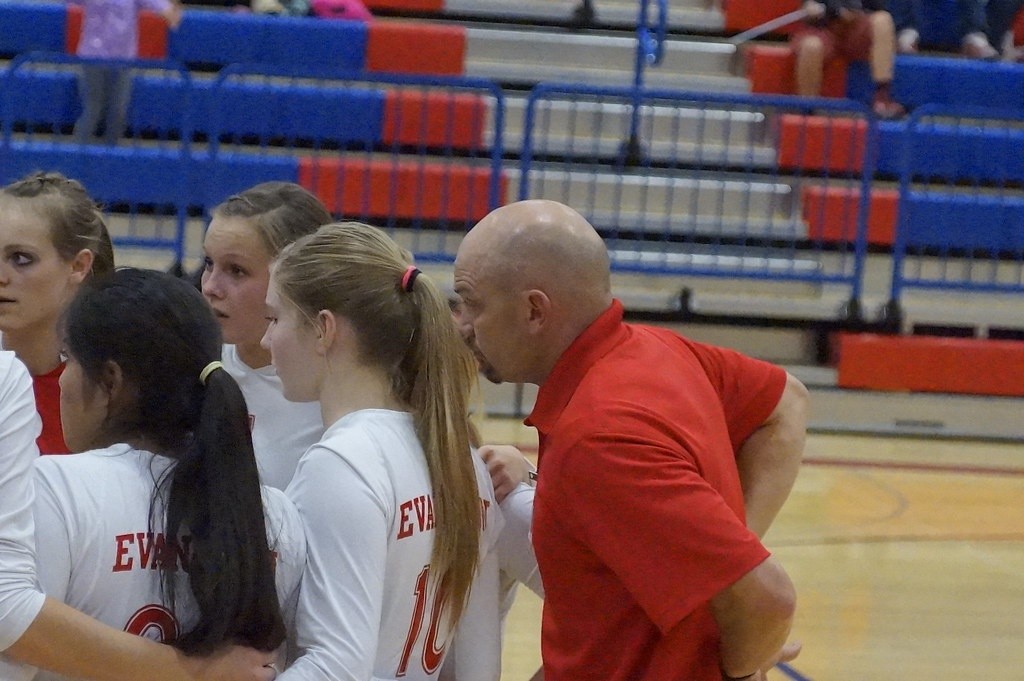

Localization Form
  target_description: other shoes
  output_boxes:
[967,42,999,60]
[899,44,918,54]
[875,100,905,119]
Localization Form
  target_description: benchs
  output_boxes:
[722,0,1024,258]
[0,0,507,228]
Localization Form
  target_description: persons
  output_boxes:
[788,0,1024,120]
[68,0,180,147]
[0,169,544,681]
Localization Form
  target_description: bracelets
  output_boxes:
[454,200,809,681]
[720,667,756,681]
